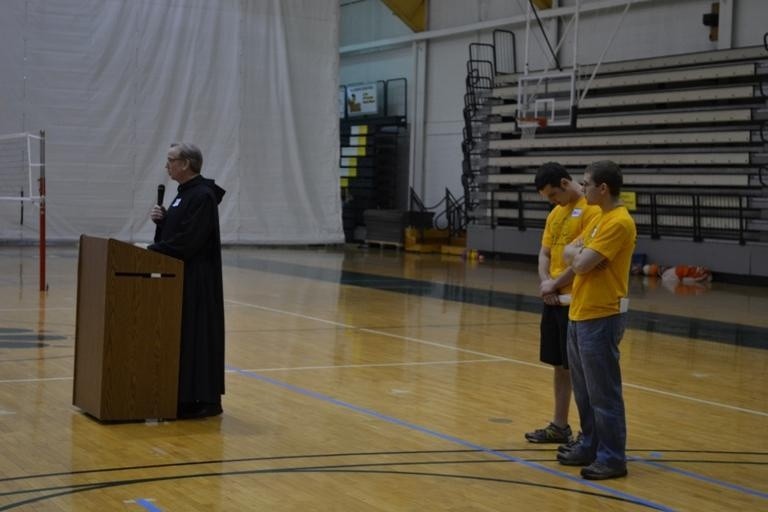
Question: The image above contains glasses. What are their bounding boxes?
[168,157,185,163]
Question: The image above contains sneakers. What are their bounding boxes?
[558,431,586,452]
[556,445,595,465]
[524,422,573,443]
[581,459,627,480]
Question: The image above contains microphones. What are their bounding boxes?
[154,184,165,224]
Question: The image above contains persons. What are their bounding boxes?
[524,161,600,453]
[134,142,227,417]
[564,159,637,478]
